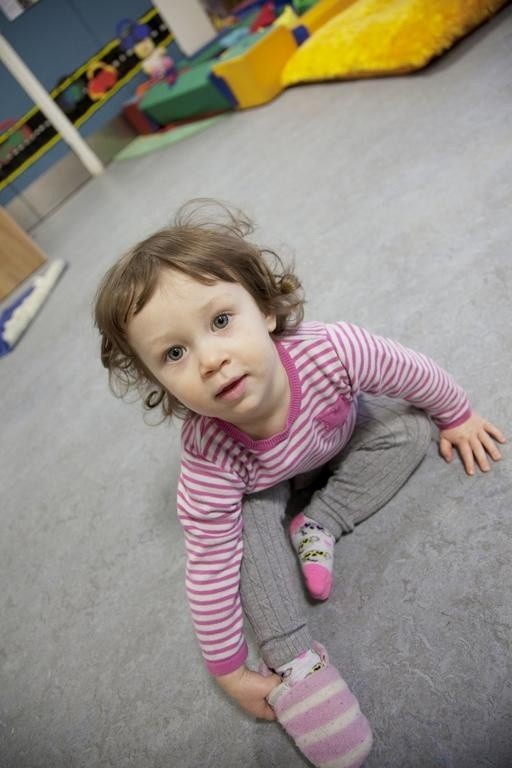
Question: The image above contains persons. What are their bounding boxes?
[83,1,358,134]
[96,196,507,768]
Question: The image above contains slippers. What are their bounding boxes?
[257,638,375,768]
